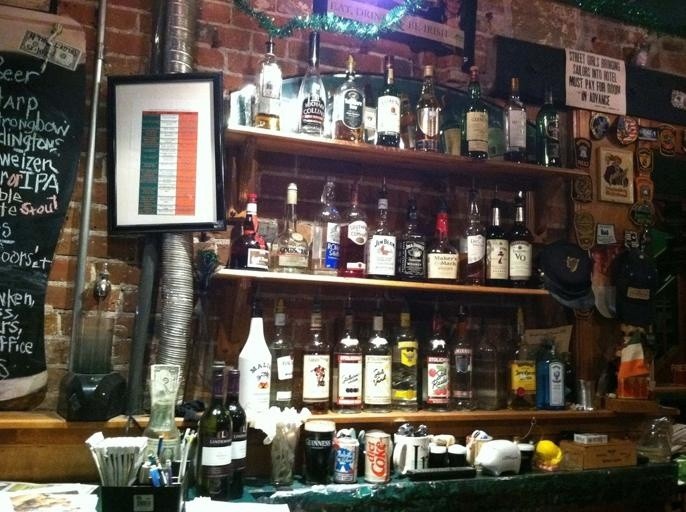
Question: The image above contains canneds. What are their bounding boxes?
[364,432,391,483]
[333,438,360,483]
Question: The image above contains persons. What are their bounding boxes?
[603,154,629,188]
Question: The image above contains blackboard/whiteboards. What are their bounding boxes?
[0,3,87,400]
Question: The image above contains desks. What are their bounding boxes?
[0,409,203,484]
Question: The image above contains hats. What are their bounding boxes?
[538,240,595,310]
[591,246,656,326]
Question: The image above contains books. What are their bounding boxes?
[84,430,150,488]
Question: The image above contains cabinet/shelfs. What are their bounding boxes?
[214,122,680,423]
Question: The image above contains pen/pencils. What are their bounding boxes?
[136,428,197,486]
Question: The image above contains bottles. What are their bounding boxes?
[426,201,460,285]
[142,382,178,461]
[224,370,248,500]
[460,66,489,159]
[439,92,462,156]
[363,298,394,414]
[269,297,295,412]
[447,445,467,468]
[397,199,426,282]
[419,299,452,412]
[199,372,232,502]
[311,175,342,276]
[450,303,474,410]
[486,198,510,288]
[458,190,487,285]
[415,64,442,153]
[534,82,562,166]
[374,53,402,147]
[392,302,419,413]
[333,296,364,415]
[508,194,532,289]
[294,31,327,136]
[474,303,579,412]
[237,304,272,417]
[231,192,269,270]
[254,38,282,130]
[362,79,377,143]
[330,51,365,143]
[398,91,417,150]
[501,75,529,161]
[366,176,398,281]
[339,175,370,278]
[293,295,333,416]
[271,183,310,274]
[517,444,535,473]
[428,446,446,469]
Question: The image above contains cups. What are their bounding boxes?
[391,433,429,477]
[302,421,335,484]
[464,436,493,465]
[267,423,299,488]
[638,417,672,463]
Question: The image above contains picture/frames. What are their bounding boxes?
[106,70,227,236]
[314,0,477,56]
